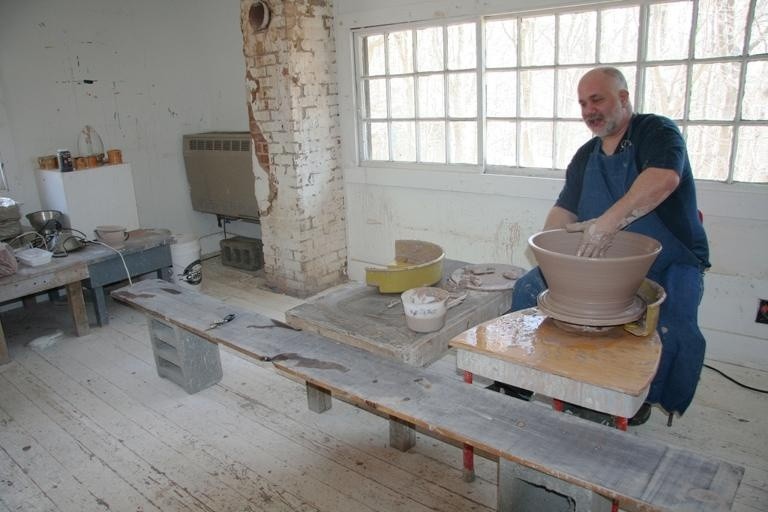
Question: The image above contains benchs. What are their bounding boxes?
[110,279,746,512]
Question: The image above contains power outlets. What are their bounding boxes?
[755,299,768,325]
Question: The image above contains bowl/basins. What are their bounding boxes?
[528,228,662,311]
[401,287,449,333]
[26,210,63,224]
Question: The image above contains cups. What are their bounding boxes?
[108,149,121,165]
[38,157,47,169]
[75,157,86,170]
[48,156,57,169]
[88,156,97,168]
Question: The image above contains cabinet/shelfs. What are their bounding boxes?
[33,165,140,245]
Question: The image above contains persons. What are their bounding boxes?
[483,66,711,428]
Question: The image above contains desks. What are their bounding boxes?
[74,229,177,328]
[285,259,513,452]
[448,306,662,512]
[0,252,91,366]
